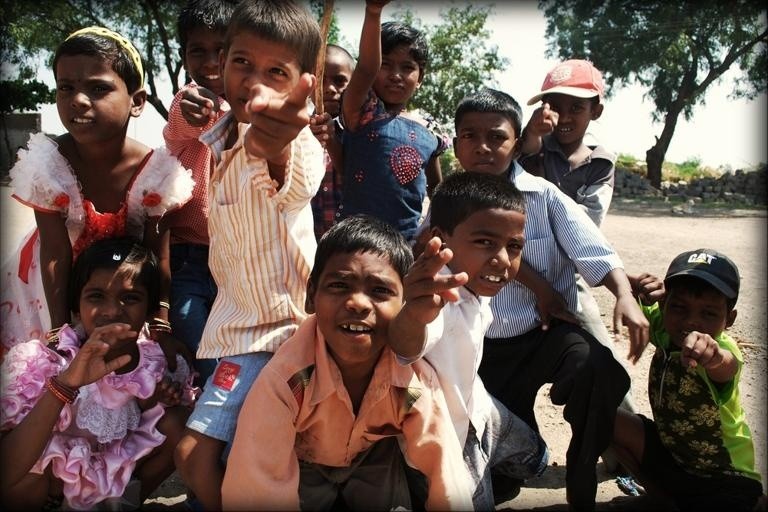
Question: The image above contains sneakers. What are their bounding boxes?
[566,452,599,506]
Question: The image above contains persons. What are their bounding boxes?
[606,249,763,511]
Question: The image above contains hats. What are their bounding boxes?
[662,246,742,307]
[525,58,604,107]
